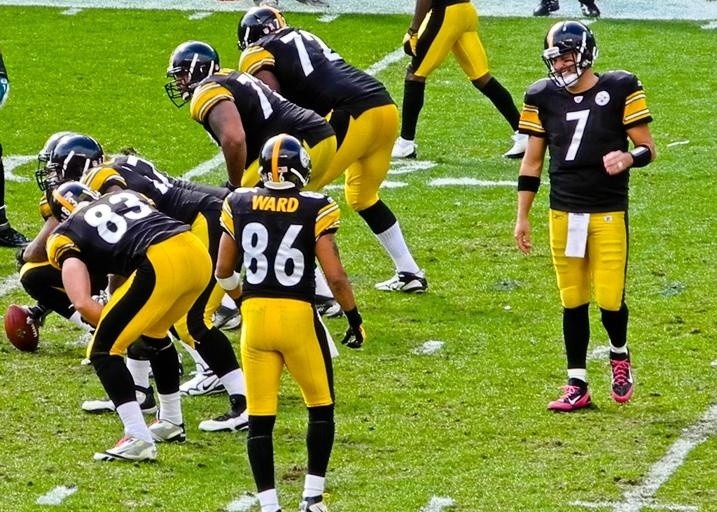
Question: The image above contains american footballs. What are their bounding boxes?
[5,305,38,351]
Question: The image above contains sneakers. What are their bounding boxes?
[178,363,227,396]
[81,385,158,415]
[391,135,418,159]
[198,394,249,432]
[375,270,428,294]
[148,410,186,444]
[298,492,330,512]
[503,130,530,159]
[0,228,30,247]
[311,294,343,319]
[533,0,600,18]
[146,354,184,378]
[547,346,634,412]
[209,304,241,330]
[93,436,157,462]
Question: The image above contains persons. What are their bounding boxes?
[37,130,233,396]
[165,41,344,321]
[515,19,655,413]
[16,136,249,434]
[1,53,28,249]
[533,0,598,17]
[238,6,428,295]
[48,169,212,460]
[214,133,366,511]
[391,1,527,158]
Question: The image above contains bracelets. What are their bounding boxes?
[518,176,540,195]
[215,271,241,292]
[629,146,652,168]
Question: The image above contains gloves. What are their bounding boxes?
[341,315,366,349]
[402,27,419,59]
[21,300,52,339]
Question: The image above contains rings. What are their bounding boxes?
[617,162,627,170]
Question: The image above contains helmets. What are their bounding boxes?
[163,5,286,108]
[541,20,600,89]
[35,131,312,225]
